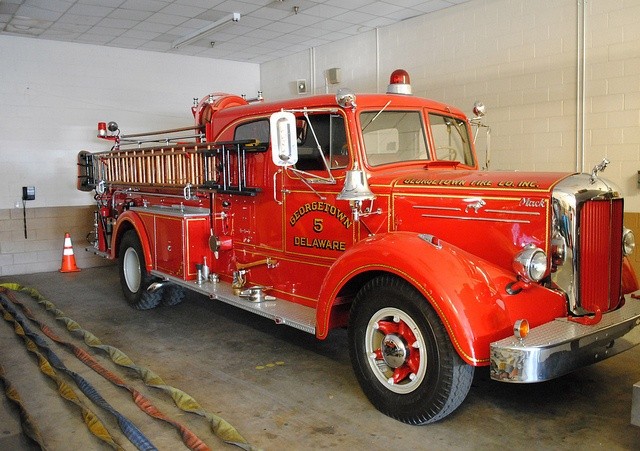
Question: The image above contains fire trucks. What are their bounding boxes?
[76,70,640,428]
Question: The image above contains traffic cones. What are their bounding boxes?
[58,233,82,274]
